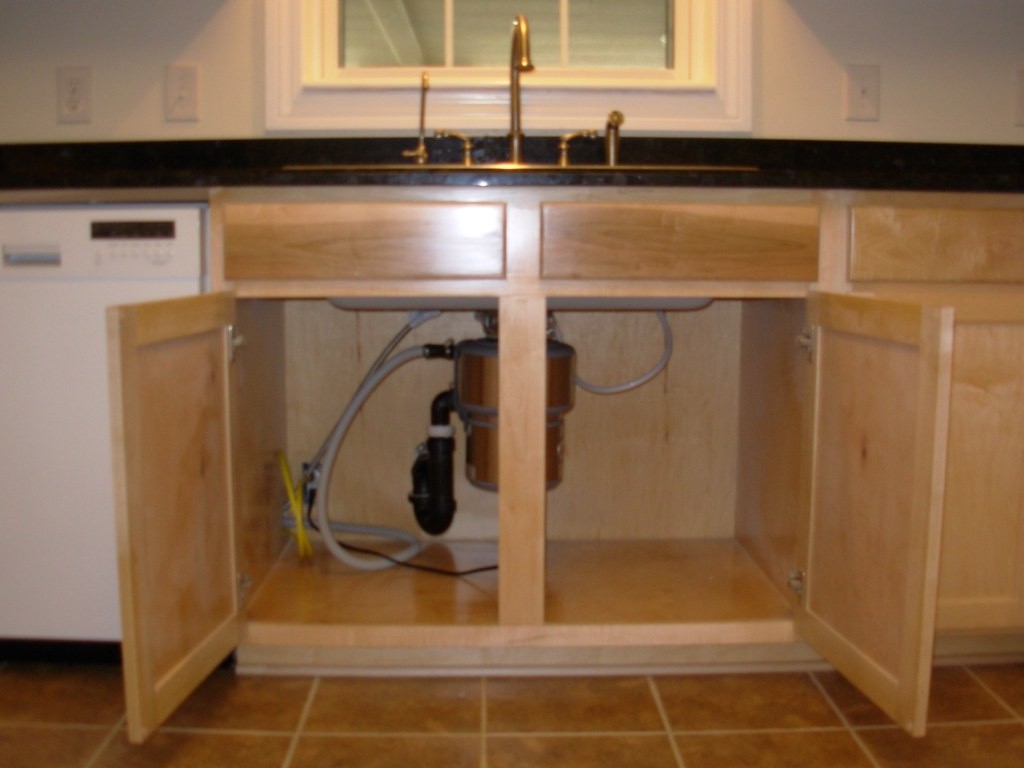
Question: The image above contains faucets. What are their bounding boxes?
[508,13,535,169]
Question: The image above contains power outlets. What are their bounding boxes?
[843,65,879,120]
[56,64,94,122]
[163,63,200,120]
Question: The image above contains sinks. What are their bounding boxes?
[285,163,761,173]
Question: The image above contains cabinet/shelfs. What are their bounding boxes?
[101,187,1024,749]
